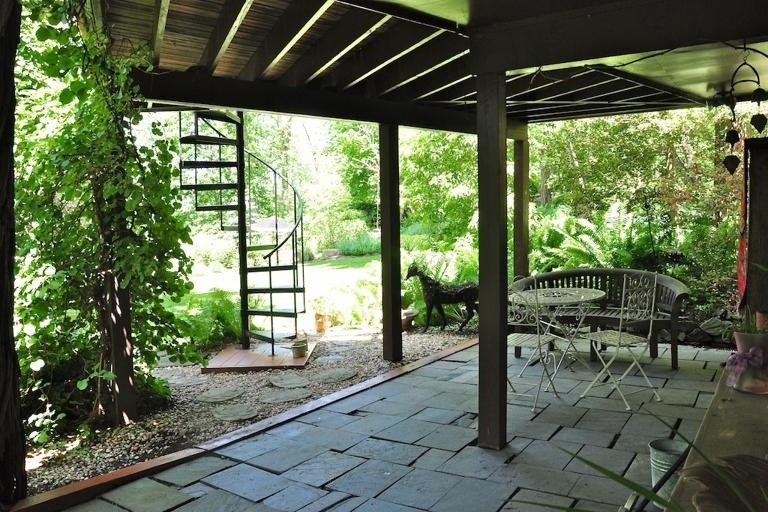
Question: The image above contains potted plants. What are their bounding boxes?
[733,304,768,365]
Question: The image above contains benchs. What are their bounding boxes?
[508,268,690,369]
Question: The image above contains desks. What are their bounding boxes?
[664,356,768,512]
[508,289,605,392]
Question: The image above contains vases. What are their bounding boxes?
[401,309,419,332]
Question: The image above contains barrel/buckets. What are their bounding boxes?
[294,338,308,351]
[647,438,690,510]
[291,342,306,358]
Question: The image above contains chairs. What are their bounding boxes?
[506,274,561,413]
[579,269,662,411]
